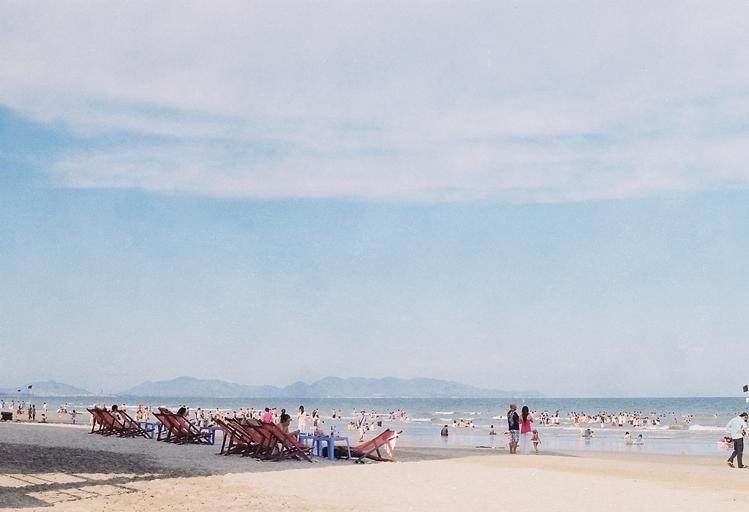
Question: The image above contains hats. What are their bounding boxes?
[739,413,748,417]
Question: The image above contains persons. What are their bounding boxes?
[489,404,560,453]
[570,410,693,443]
[721,413,749,468]
[90,403,284,439]
[440,419,473,437]
[1,401,83,424]
[278,406,407,450]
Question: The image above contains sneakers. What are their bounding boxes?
[727,461,749,469]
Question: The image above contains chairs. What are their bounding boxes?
[212,414,352,465]
[337,428,399,464]
[88,408,158,441]
[152,408,216,445]
